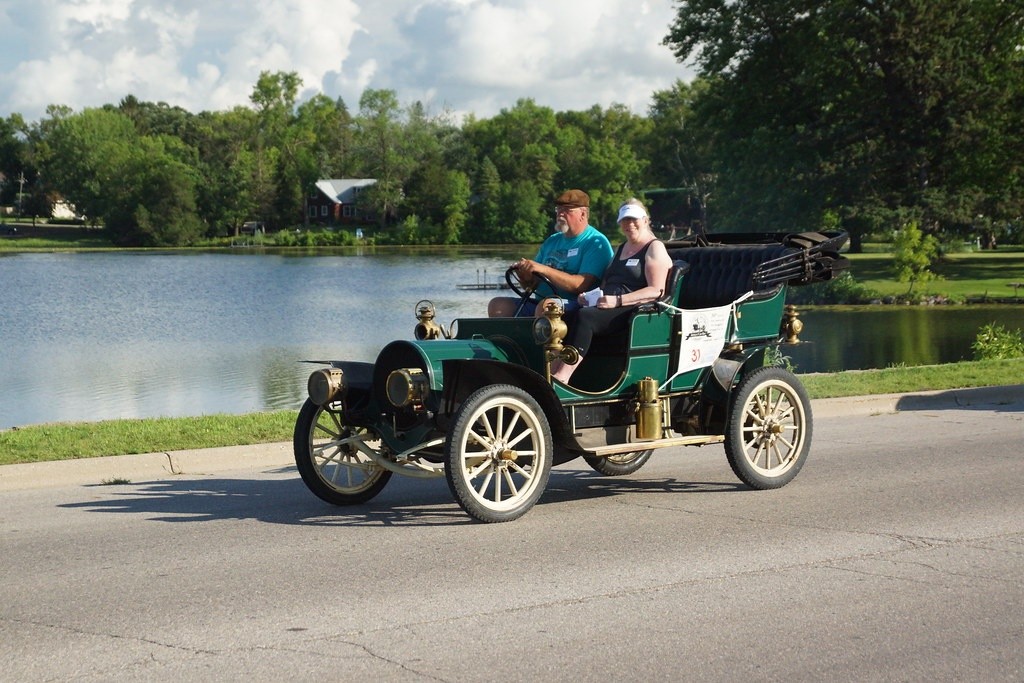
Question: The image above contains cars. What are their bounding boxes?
[289,226,851,525]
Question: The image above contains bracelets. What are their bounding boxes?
[616,295,621,307]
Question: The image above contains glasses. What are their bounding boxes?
[554,207,585,213]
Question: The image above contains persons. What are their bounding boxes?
[489,189,614,319]
[538,198,672,387]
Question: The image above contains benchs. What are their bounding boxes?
[590,259,687,389]
[666,242,784,311]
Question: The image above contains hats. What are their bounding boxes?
[553,189,590,207]
[616,205,646,223]
